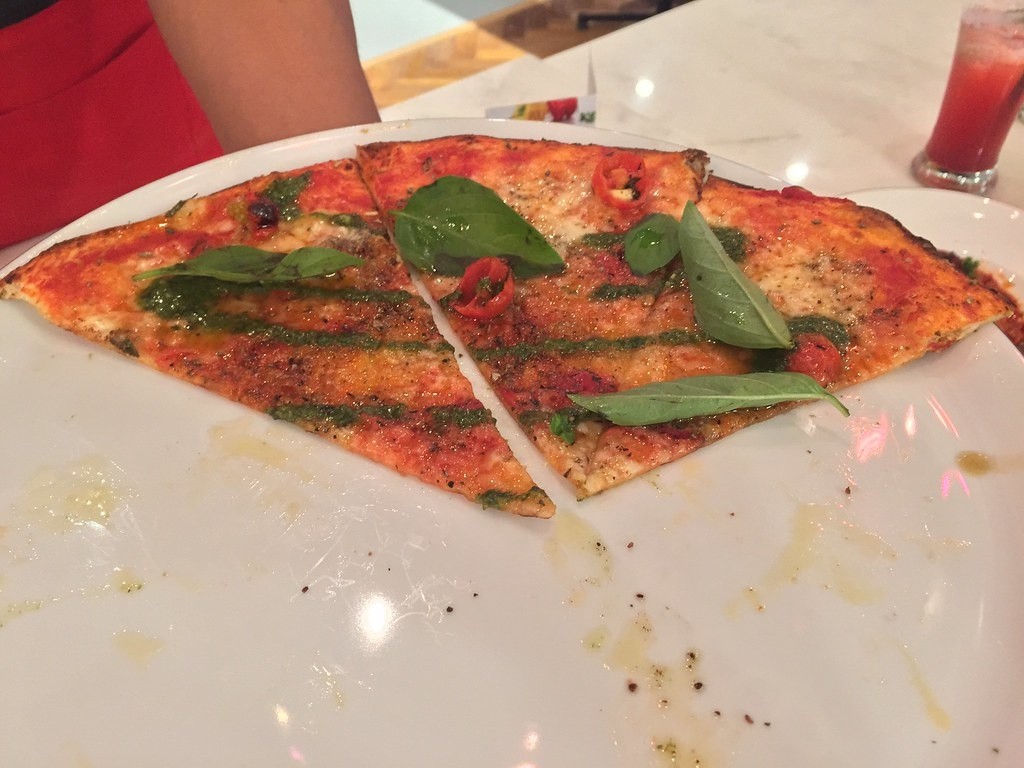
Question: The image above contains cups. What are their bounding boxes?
[912,1,1023,198]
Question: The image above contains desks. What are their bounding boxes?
[374,0,1024,354]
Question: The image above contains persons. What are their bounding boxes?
[1,1,382,248]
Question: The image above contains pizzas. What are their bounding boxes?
[0,132,1019,518]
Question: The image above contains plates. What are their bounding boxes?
[3,118,1024,768]
[827,189,1024,313]
